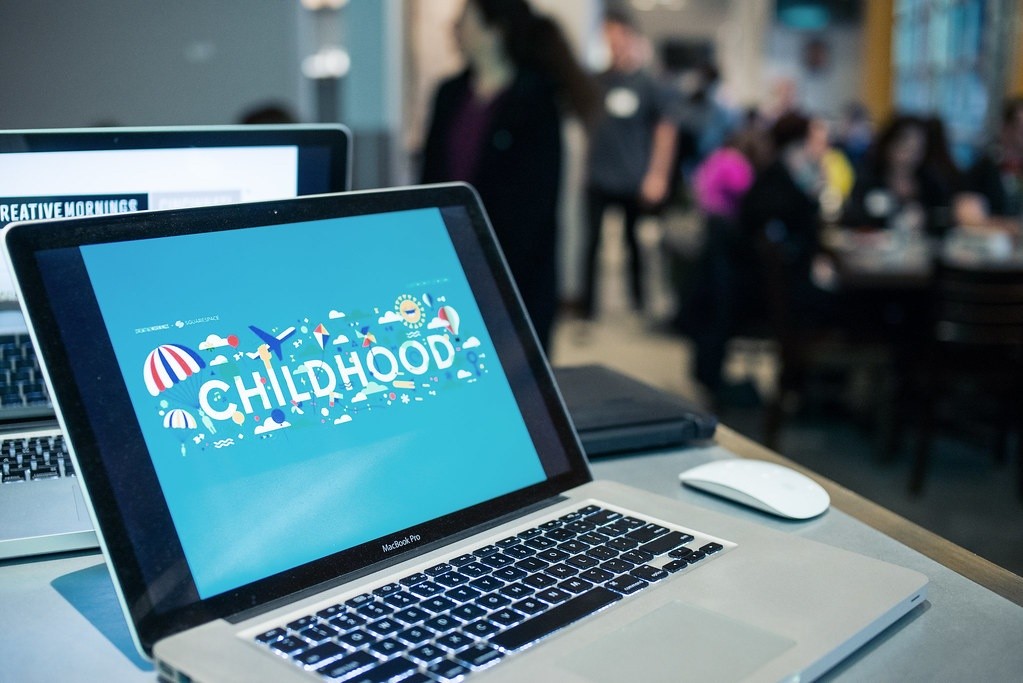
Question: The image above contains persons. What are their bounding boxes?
[411,0,567,363]
[671,65,1022,433]
[558,7,683,320]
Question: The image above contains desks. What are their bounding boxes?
[0,358,1023,683]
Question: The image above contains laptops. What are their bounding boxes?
[0,121,353,561]
[1,183,928,683]
[552,362,717,456]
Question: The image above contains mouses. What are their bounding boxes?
[678,457,831,522]
